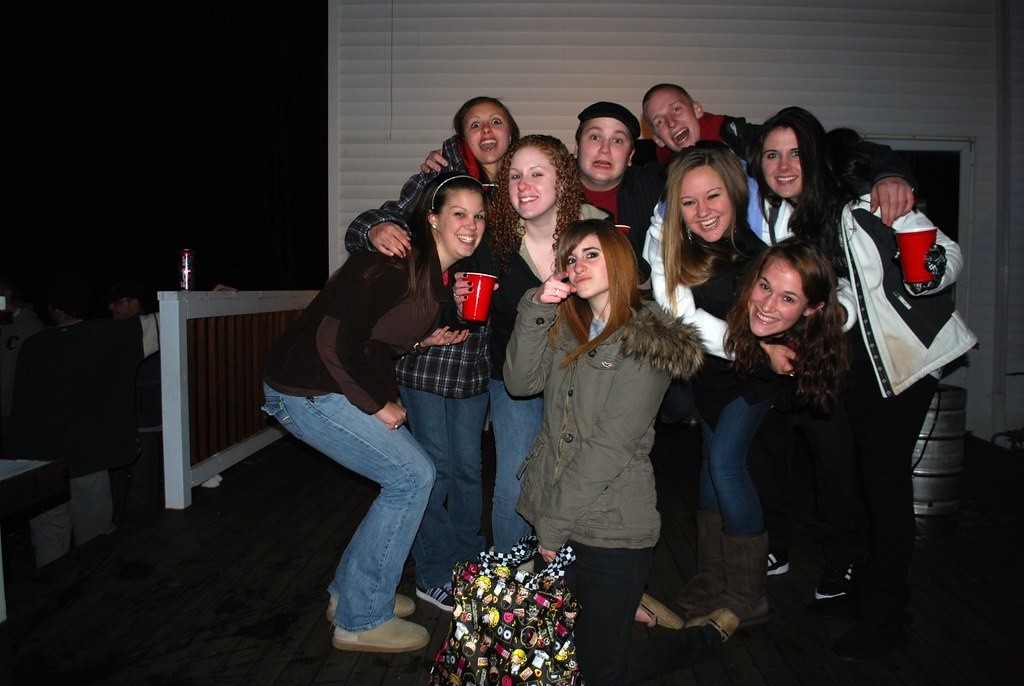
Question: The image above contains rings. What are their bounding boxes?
[790,372,794,376]
[446,343,450,345]
[395,425,399,428]
[556,289,559,296]
[454,293,457,297]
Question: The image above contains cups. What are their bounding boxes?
[461,272,498,323]
[894,227,938,282]
[615,225,631,235]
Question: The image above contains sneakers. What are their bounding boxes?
[767,553,790,574]
[416,580,453,611]
[815,562,856,600]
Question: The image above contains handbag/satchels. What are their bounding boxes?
[427,535,585,686]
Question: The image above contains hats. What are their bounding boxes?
[578,101,641,141]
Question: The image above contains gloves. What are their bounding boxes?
[895,243,947,293]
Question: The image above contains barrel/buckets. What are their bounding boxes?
[910,384,968,517]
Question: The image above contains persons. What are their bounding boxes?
[0,279,239,569]
[262,172,486,653]
[422,100,672,301]
[346,98,523,613]
[643,83,915,227]
[504,219,740,686]
[750,107,978,660]
[641,149,860,627]
[486,133,613,552]
[722,237,861,602]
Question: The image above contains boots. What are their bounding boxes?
[684,528,773,624]
[675,510,728,608]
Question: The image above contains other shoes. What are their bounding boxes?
[327,591,416,622]
[333,615,429,654]
[831,623,907,659]
[806,593,866,618]
[639,593,683,630]
[687,607,740,641]
